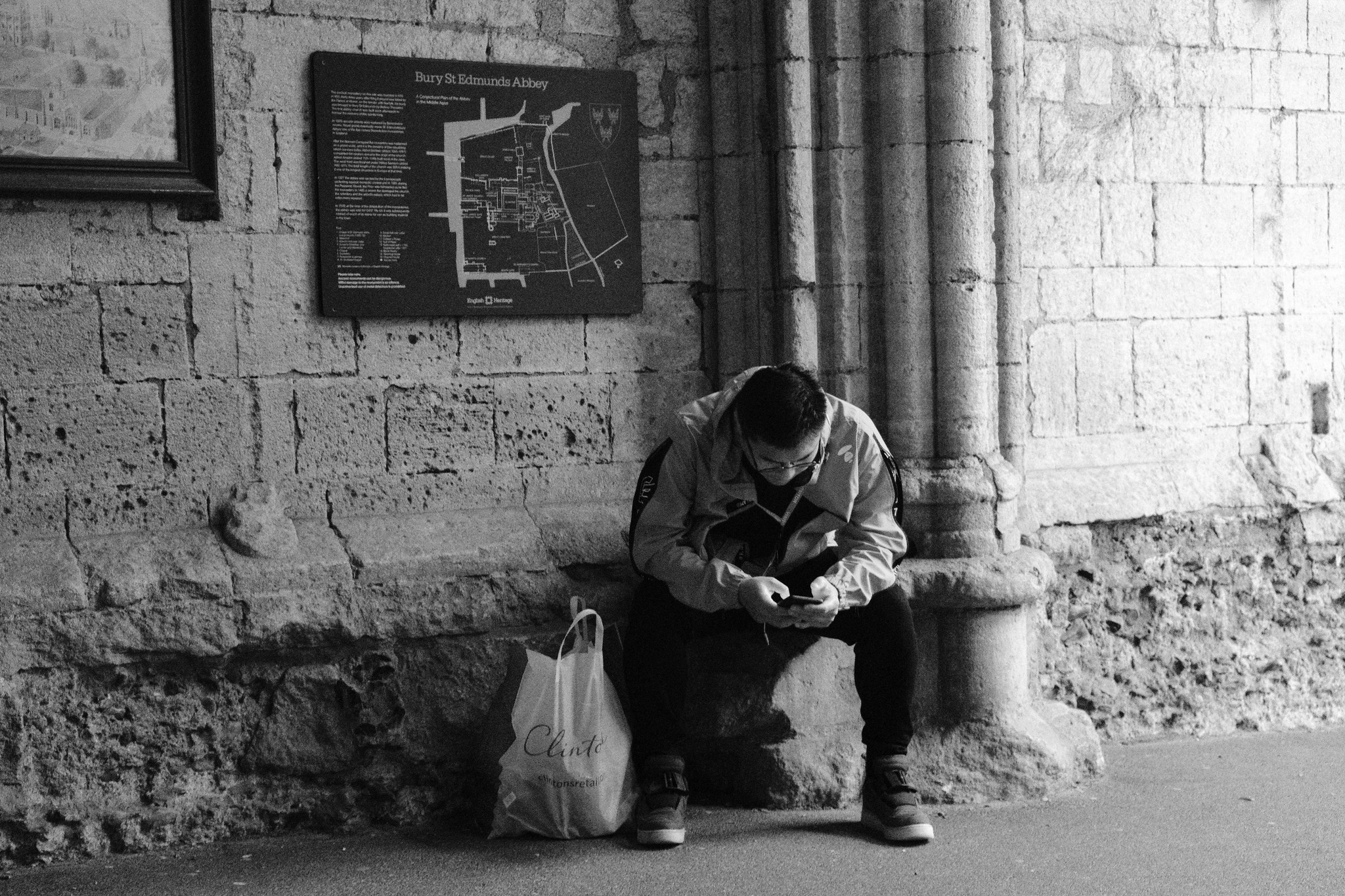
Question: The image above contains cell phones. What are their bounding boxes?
[777,596,823,608]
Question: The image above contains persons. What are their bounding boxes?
[619,362,935,844]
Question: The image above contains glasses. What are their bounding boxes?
[742,437,822,475]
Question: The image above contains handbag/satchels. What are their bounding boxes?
[485,597,642,841]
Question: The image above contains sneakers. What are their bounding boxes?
[859,766,935,842]
[633,754,690,847]
